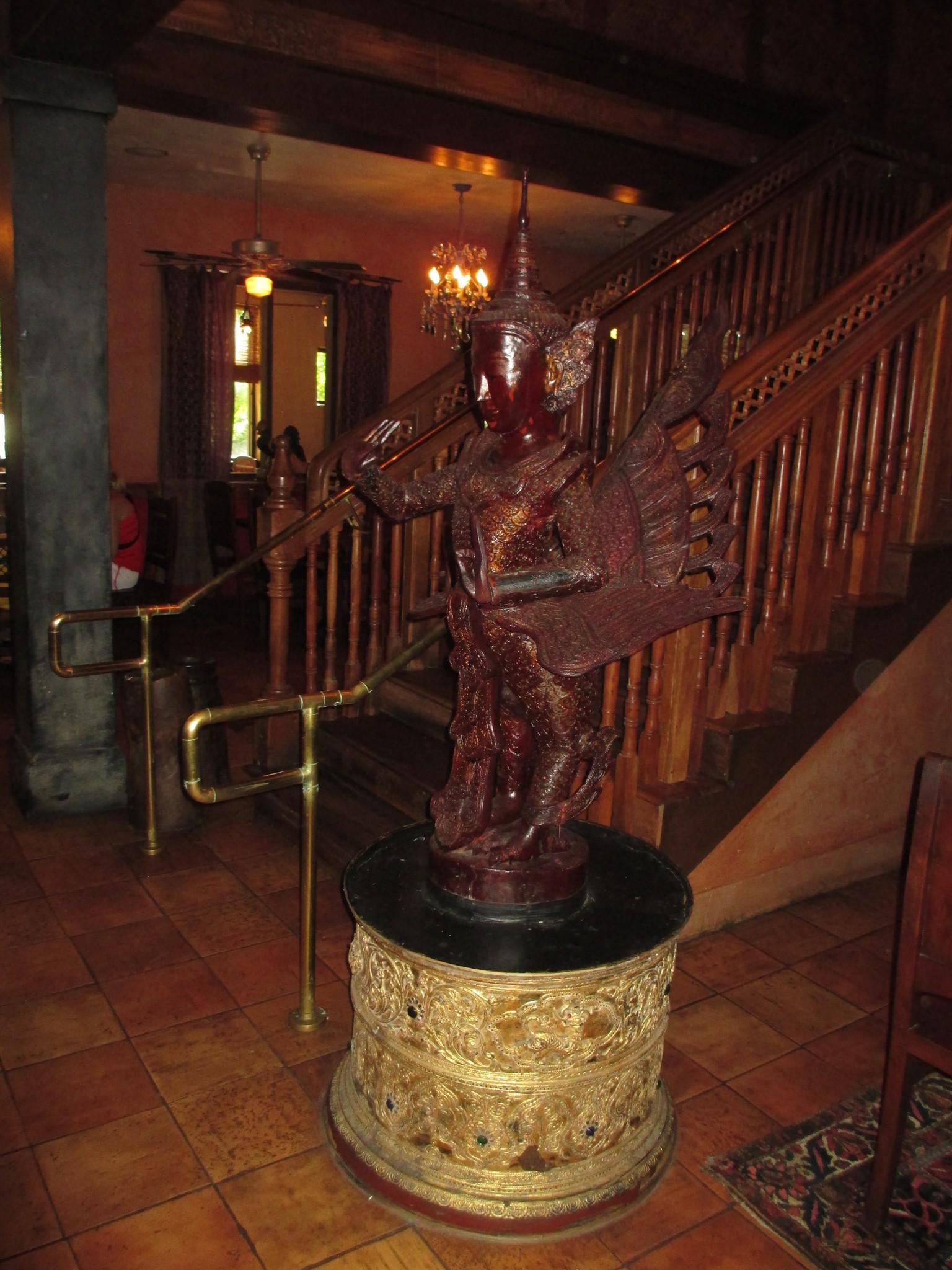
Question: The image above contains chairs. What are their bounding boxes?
[203,478,269,641]
[860,750,952,1240]
[111,482,179,666]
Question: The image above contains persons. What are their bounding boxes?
[110,487,141,589]
[340,288,607,859]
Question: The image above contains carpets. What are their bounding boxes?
[701,1060,952,1270]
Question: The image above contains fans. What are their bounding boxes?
[139,145,403,295]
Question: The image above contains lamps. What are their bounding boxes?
[243,268,274,299]
[419,183,490,353]
